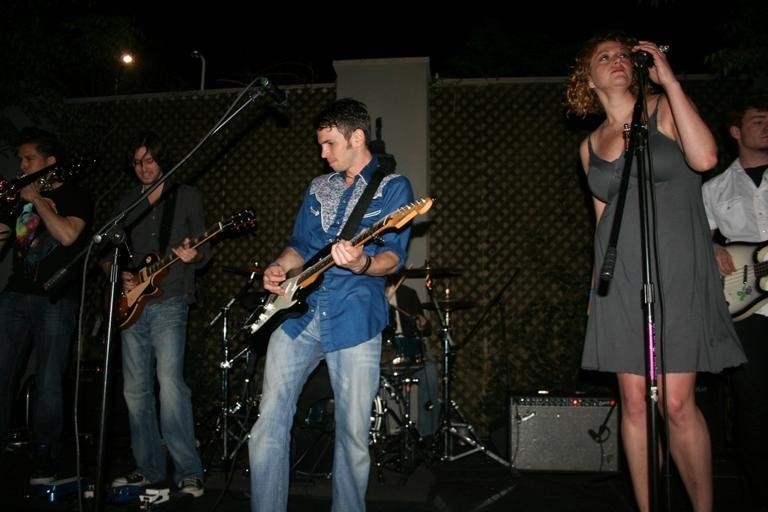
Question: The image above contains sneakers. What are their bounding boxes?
[29,472,81,486]
[112,471,151,487]
[177,478,204,497]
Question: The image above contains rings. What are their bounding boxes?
[656,43,671,56]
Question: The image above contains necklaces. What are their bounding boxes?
[603,109,634,135]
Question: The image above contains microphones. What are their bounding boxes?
[261,78,291,108]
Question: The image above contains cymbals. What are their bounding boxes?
[422,300,477,310]
[402,268,461,279]
[223,266,265,275]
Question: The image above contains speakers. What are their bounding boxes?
[507,393,621,476]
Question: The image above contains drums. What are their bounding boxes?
[379,379,419,434]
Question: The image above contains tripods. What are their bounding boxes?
[194,308,252,474]
[421,308,512,475]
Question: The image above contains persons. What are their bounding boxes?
[97,133,216,497]
[563,28,750,511]
[383,275,432,360]
[1,126,87,488]
[702,95,768,512]
[244,95,413,510]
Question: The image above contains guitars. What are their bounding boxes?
[104,211,257,331]
[719,241,768,322]
[248,197,433,334]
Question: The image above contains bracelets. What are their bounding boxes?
[353,253,372,276]
